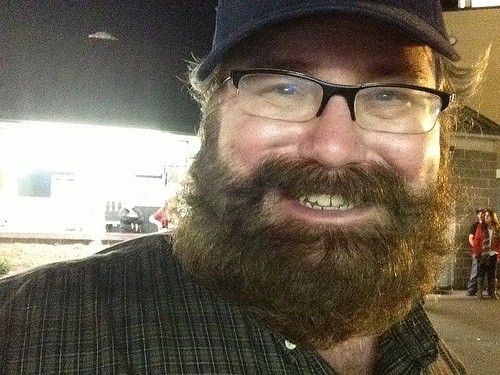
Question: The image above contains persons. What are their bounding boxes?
[468,208,499,300]
[0,0,469,375]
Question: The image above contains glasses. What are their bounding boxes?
[220,68,457,134]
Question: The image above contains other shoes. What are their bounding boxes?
[477,294,483,299]
[488,293,495,298]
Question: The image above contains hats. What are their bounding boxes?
[476,207,484,212]
[196,0,462,83]
